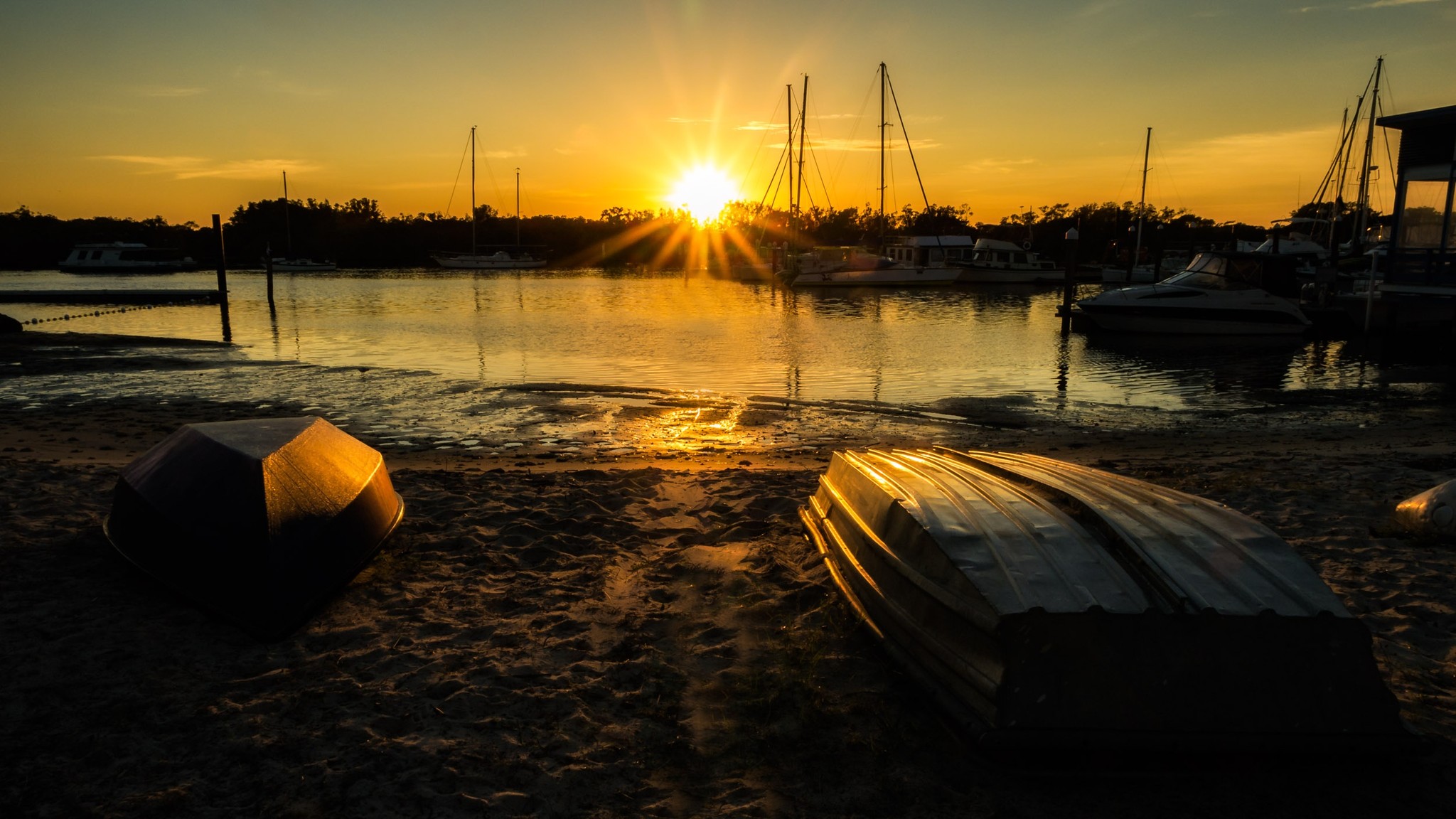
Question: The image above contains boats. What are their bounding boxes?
[1066,250,1315,341]
[57,242,198,275]
[798,443,1403,765]
[102,417,407,609]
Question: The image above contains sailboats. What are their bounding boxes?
[712,61,1070,289]
[260,170,336,271]
[1078,56,1439,300]
[429,127,547,268]
